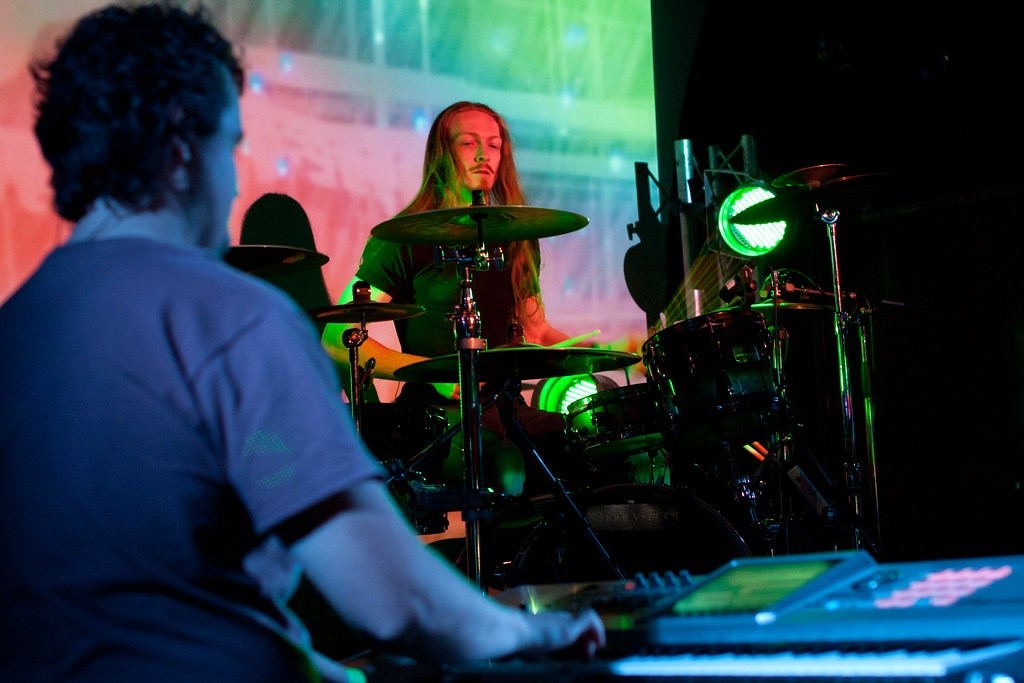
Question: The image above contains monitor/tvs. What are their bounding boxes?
[671,559,840,613]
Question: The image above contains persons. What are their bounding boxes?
[321,101,599,513]
[0,1,606,683]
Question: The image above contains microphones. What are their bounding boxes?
[759,269,787,298]
[719,261,756,303]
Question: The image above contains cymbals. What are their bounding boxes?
[370,203,589,244]
[391,342,643,390]
[306,300,425,324]
[729,162,888,226]
[222,244,330,270]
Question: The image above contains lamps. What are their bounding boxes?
[539,375,621,436]
[718,179,787,258]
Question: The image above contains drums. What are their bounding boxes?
[567,382,662,456]
[549,430,672,486]
[497,479,751,585]
[642,308,784,448]
